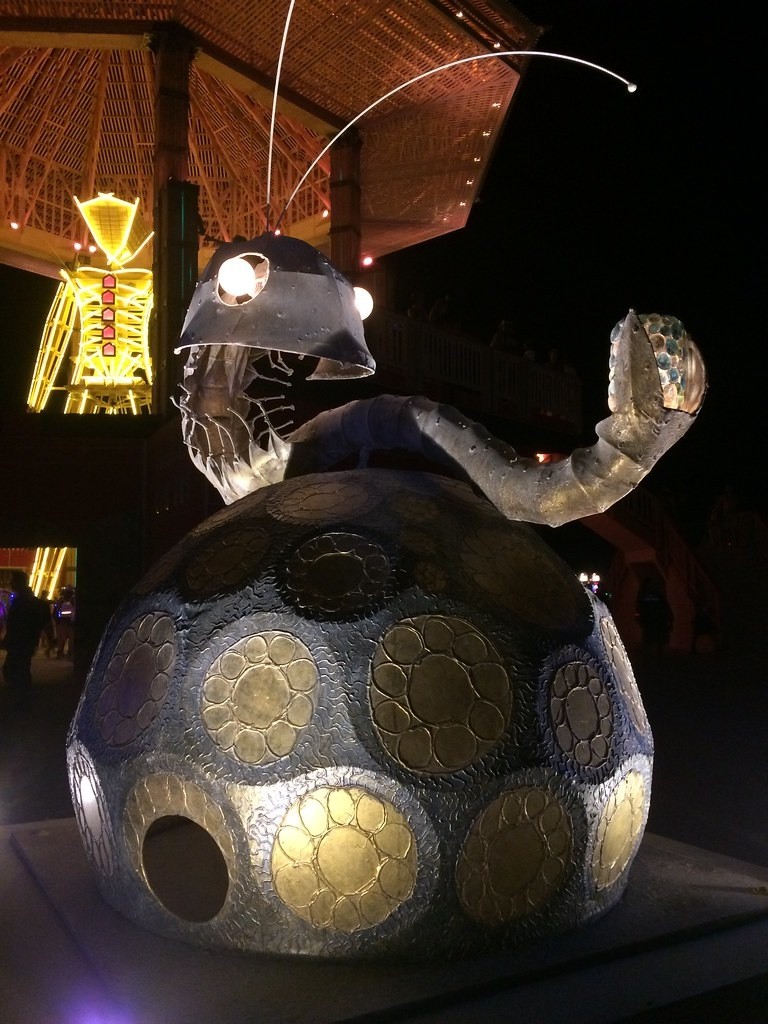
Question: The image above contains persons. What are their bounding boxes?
[0,570,75,691]
